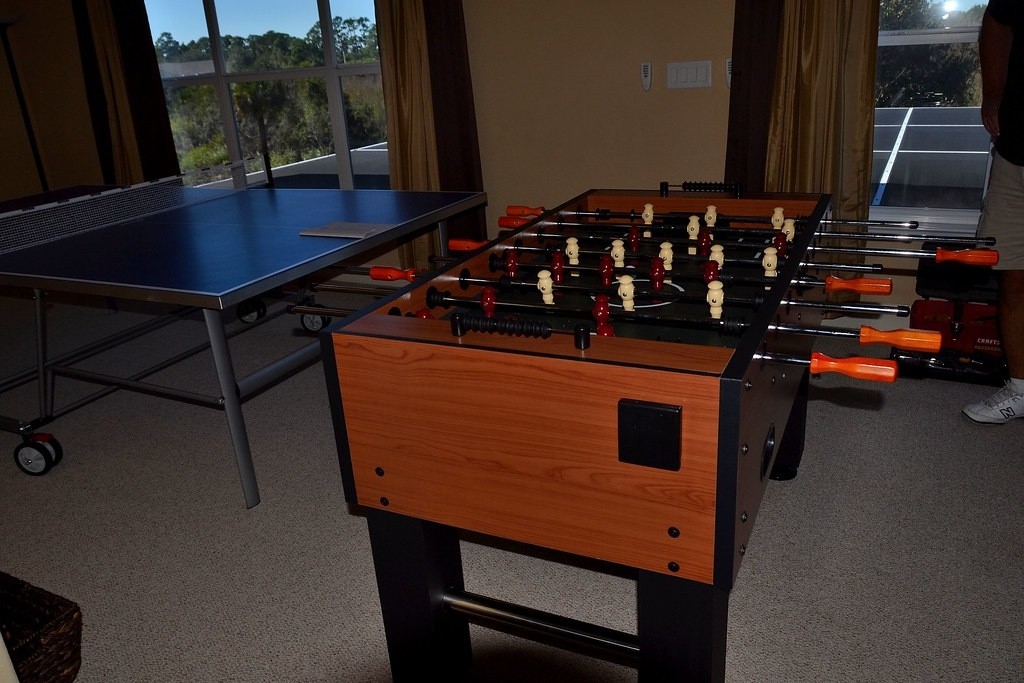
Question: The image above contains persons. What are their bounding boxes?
[962,0,1024,423]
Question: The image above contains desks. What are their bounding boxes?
[0,183,489,510]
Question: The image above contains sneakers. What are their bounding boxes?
[961,380,1024,424]
